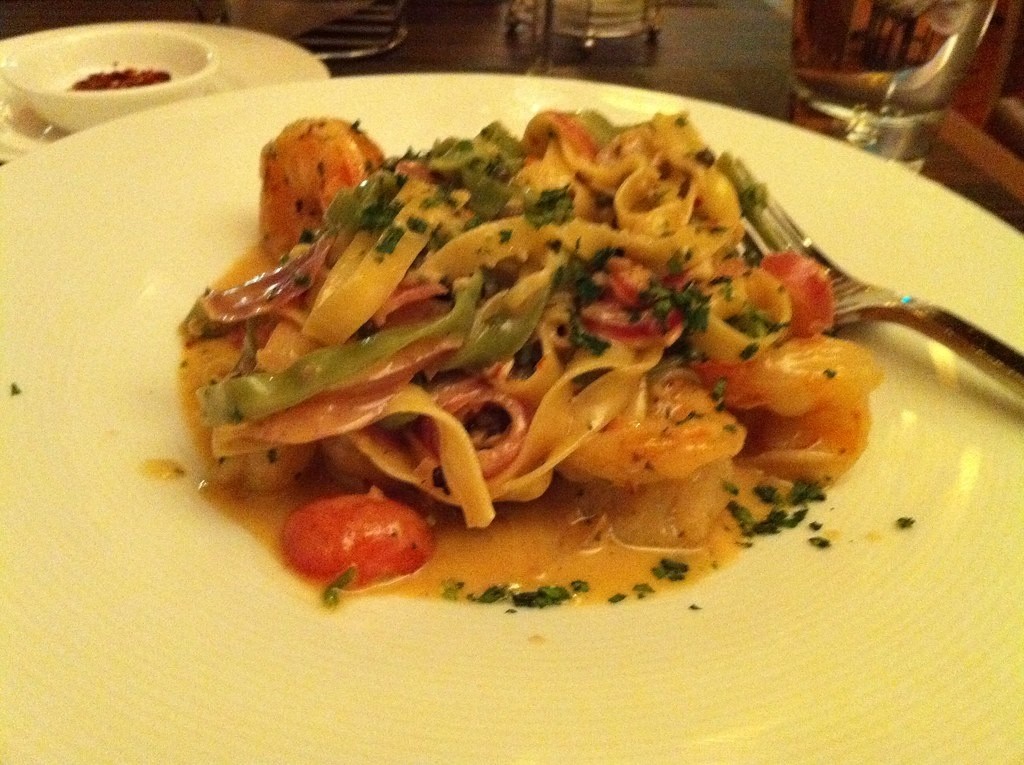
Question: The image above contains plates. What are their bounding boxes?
[0,20,332,164]
[0,73,1024,765]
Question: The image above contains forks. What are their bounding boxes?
[741,197,1024,396]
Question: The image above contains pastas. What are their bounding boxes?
[179,106,883,608]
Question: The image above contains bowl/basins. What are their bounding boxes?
[5,29,221,133]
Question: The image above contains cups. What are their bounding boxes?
[789,0,997,125]
